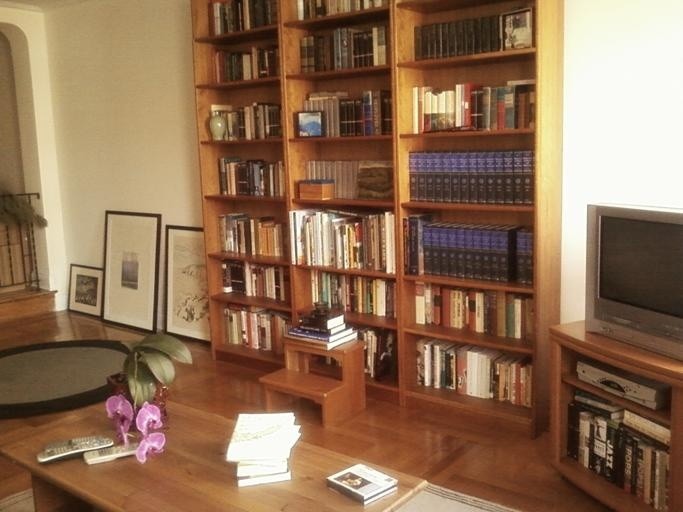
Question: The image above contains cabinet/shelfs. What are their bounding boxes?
[253,334,367,429]
[390,1,565,436]
[279,0,398,409]
[543,321,683,511]
[189,1,289,365]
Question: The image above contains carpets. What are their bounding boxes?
[0,339,132,420]
[0,393,466,512]
[0,483,526,512]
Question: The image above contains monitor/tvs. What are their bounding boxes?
[586,204,683,361]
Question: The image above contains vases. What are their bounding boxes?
[106,372,168,425]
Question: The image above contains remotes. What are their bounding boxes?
[84,443,137,464]
[37,437,114,462]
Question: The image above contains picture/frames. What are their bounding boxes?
[67,263,104,318]
[164,224,211,345]
[100,211,162,336]
[499,7,535,51]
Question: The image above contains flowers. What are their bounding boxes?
[105,335,192,464]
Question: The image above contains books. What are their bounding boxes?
[413,79,536,134]
[326,463,398,506]
[414,14,502,61]
[288,208,396,275]
[408,150,534,205]
[224,303,292,355]
[208,0,276,35]
[309,269,397,320]
[219,157,285,196]
[210,47,279,84]
[281,310,360,351]
[210,103,281,140]
[414,282,534,345]
[226,411,302,488]
[415,338,532,408]
[217,213,286,258]
[300,28,388,74]
[566,389,671,510]
[303,90,391,137]
[352,326,396,381]
[222,259,290,305]
[306,159,392,199]
[296,0,389,20]
[402,214,534,285]
